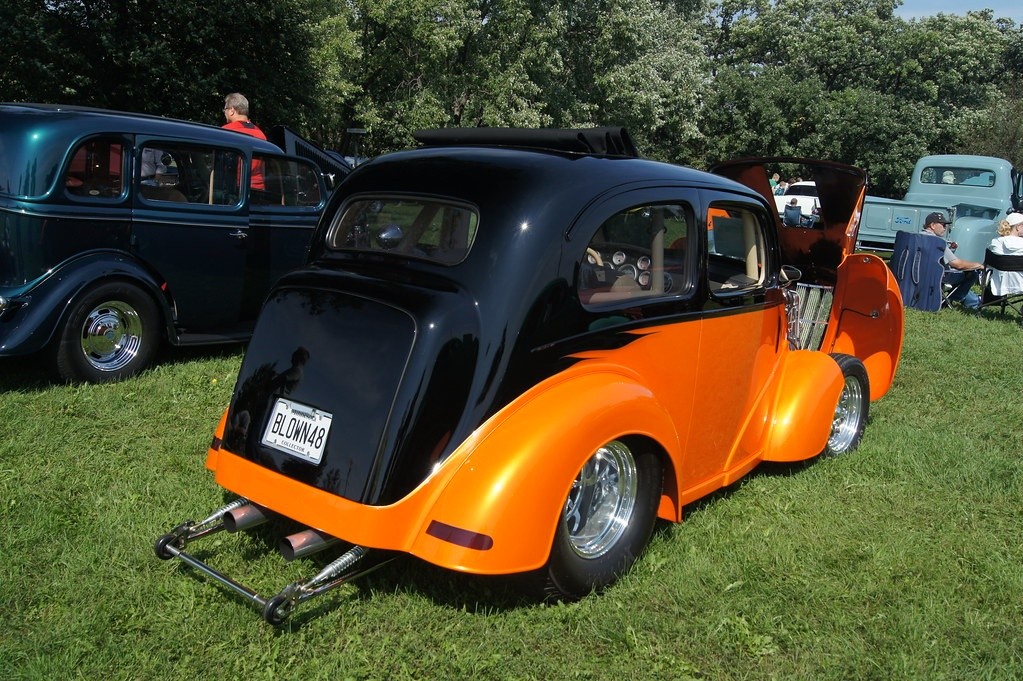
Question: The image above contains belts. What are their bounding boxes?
[140,175,155,181]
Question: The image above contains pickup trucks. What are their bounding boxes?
[855,154,1015,274]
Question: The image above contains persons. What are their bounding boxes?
[220,93,268,190]
[918,211,984,309]
[982,212,1023,315]
[131,143,169,188]
[783,198,825,228]
[66,174,113,198]
[769,173,803,196]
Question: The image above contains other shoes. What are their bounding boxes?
[1018,306,1023,316]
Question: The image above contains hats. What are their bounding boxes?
[925,212,954,225]
[1005,212,1023,226]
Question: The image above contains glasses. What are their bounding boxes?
[222,107,233,113]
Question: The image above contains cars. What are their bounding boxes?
[774,179,824,219]
[0,103,337,388]
[214,141,907,590]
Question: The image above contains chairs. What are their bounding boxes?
[975,247,1023,319]
[888,230,983,313]
